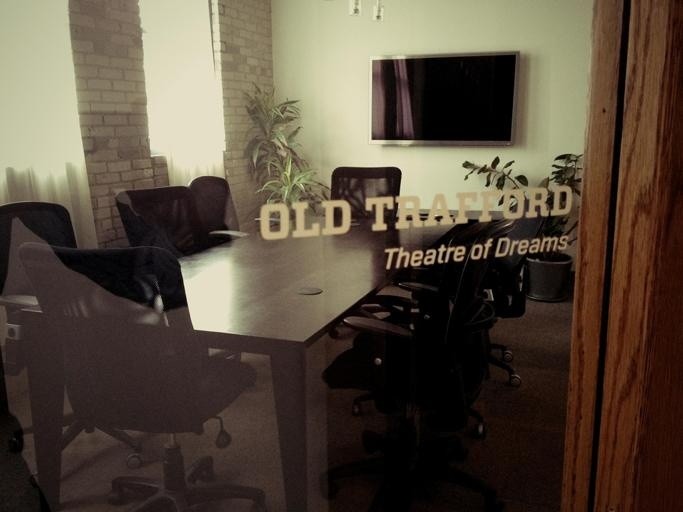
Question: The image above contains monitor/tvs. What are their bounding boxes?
[368,50,520,146]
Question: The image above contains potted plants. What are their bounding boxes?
[461,153,584,303]
